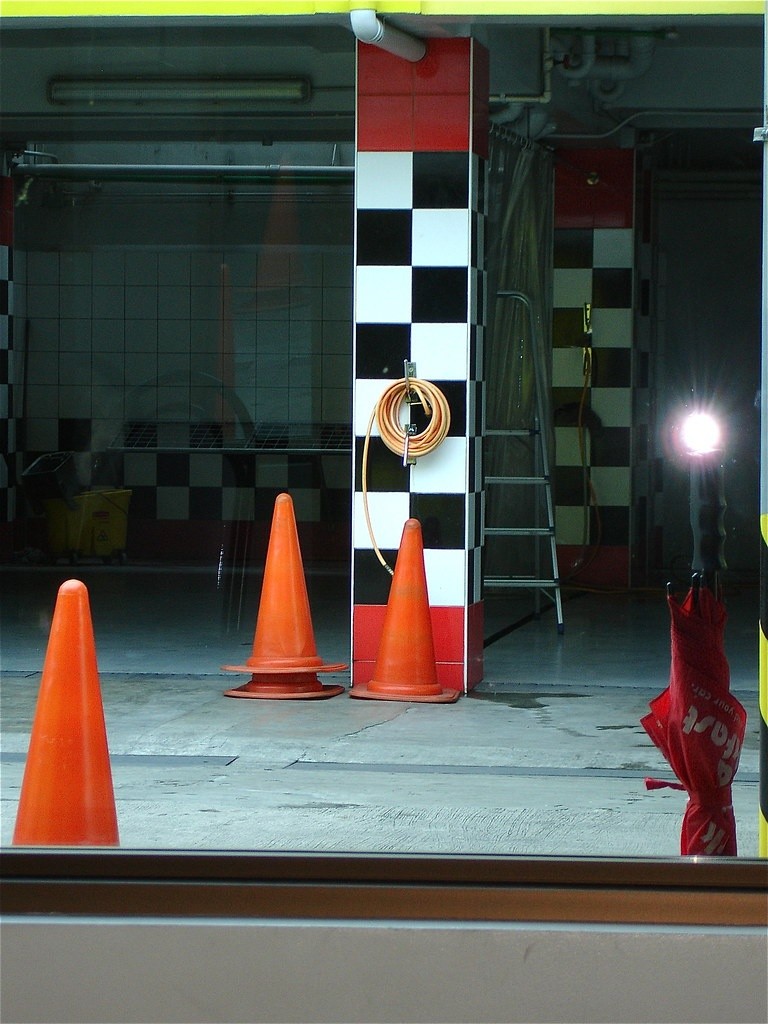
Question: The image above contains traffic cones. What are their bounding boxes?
[8,578,120,849]
[349,516,464,706]
[219,493,348,701]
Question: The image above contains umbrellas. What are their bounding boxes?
[644,433,747,857]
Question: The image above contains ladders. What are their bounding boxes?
[484,291,564,635]
